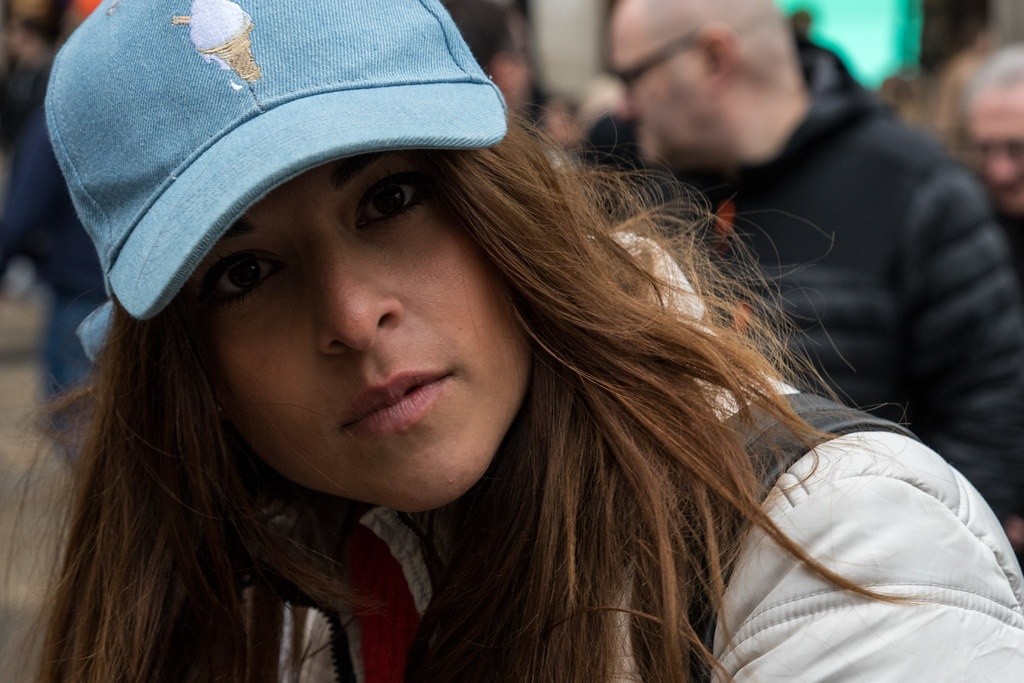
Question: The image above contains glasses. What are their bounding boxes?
[608,39,697,102]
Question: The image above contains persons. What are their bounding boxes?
[0,0,1024,683]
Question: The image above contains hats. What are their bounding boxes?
[46,0,507,322]
